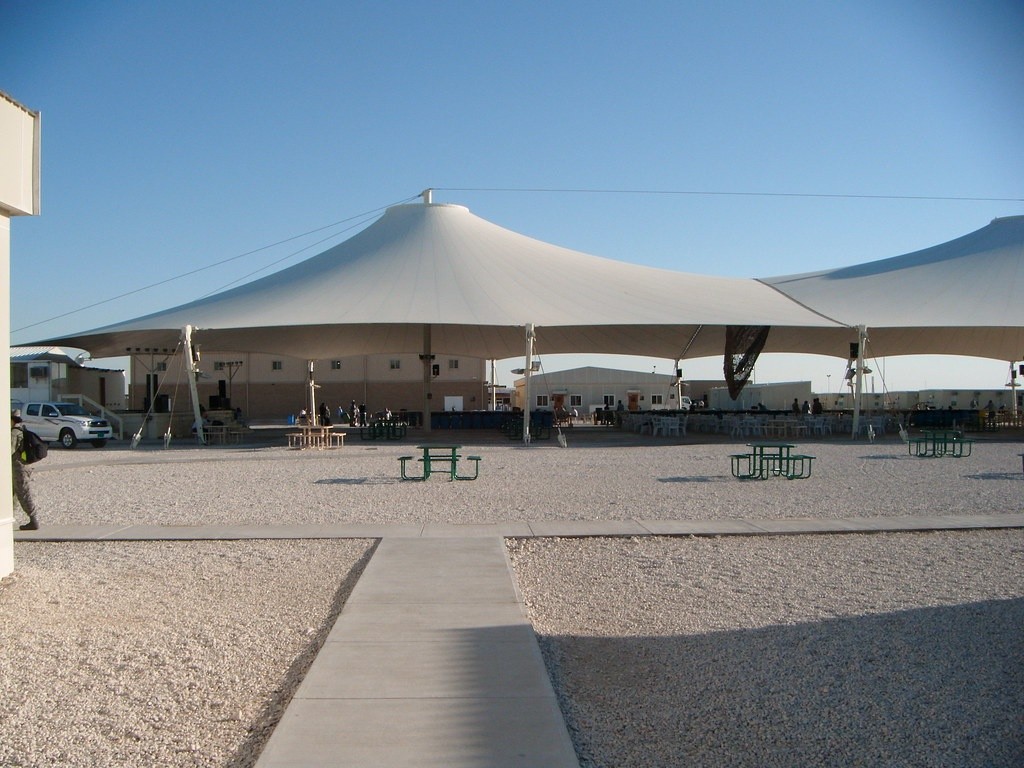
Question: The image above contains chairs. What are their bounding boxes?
[593,408,1024,438]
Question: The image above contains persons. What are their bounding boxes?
[384,408,392,420]
[757,403,766,410]
[700,400,704,408]
[349,399,366,427]
[299,406,307,418]
[916,404,920,410]
[984,399,996,427]
[191,410,216,446]
[604,403,609,410]
[617,400,624,411]
[802,401,810,413]
[926,404,930,410]
[319,403,331,426]
[812,398,822,414]
[571,407,578,415]
[11,408,39,530]
[998,404,1006,414]
[792,398,800,411]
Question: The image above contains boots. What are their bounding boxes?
[19,517,40,530]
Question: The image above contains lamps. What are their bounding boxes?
[845,369,856,379]
[432,364,440,379]
[511,369,525,375]
[1012,364,1024,379]
[863,366,873,374]
[669,382,679,387]
[681,383,689,387]
[530,361,541,372]
[418,354,436,363]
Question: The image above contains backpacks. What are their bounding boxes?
[11,425,47,465]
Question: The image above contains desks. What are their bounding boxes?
[298,425,334,451]
[919,429,961,458]
[369,419,398,441]
[768,419,801,439]
[747,442,796,480]
[417,442,461,483]
[203,425,242,445]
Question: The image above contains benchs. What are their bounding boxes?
[397,456,414,481]
[907,438,974,459]
[466,456,483,481]
[357,425,408,442]
[286,433,347,450]
[417,454,461,481]
[193,432,253,445]
[727,454,750,479]
[791,453,817,480]
[746,453,783,480]
[759,456,803,480]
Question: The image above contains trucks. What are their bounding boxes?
[681,396,692,412]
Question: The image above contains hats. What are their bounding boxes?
[11,408,22,417]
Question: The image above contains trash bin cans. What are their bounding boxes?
[341,412,351,423]
[287,413,295,425]
[160,395,169,413]
[209,395,220,410]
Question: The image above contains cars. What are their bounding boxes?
[17,402,114,447]
